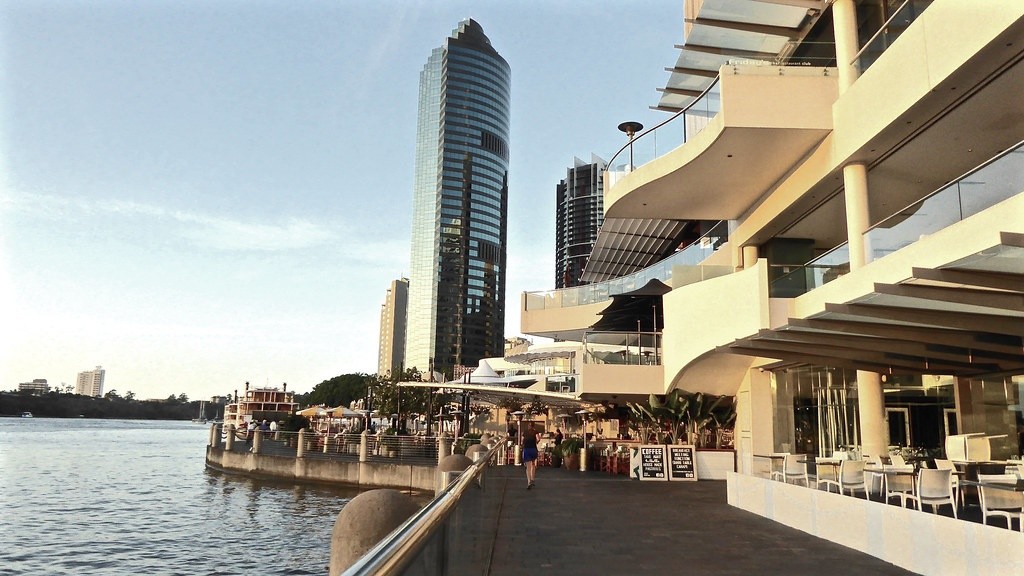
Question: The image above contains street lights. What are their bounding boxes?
[575,409,593,450]
[448,410,462,440]
[510,410,528,445]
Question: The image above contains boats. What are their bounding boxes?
[21,411,34,418]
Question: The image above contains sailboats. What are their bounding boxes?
[191,400,224,425]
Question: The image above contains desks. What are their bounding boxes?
[754,454,821,486]
[863,467,965,511]
[796,459,875,494]
[952,460,1023,518]
[641,351,654,365]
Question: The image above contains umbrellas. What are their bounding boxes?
[325,405,363,424]
[294,405,329,425]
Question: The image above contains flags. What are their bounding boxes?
[565,265,570,288]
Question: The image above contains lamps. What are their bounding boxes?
[881,368,896,384]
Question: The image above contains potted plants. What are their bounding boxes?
[552,433,593,470]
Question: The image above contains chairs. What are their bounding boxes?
[507,445,1024,536]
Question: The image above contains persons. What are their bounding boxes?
[352,424,358,432]
[506,424,518,437]
[416,432,427,436]
[549,432,556,439]
[372,421,376,431]
[309,423,322,451]
[269,420,277,439]
[521,421,540,490]
[555,429,563,446]
[248,419,268,441]
[334,423,351,452]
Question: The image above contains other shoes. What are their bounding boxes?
[530,480,536,486]
[527,483,533,490]
